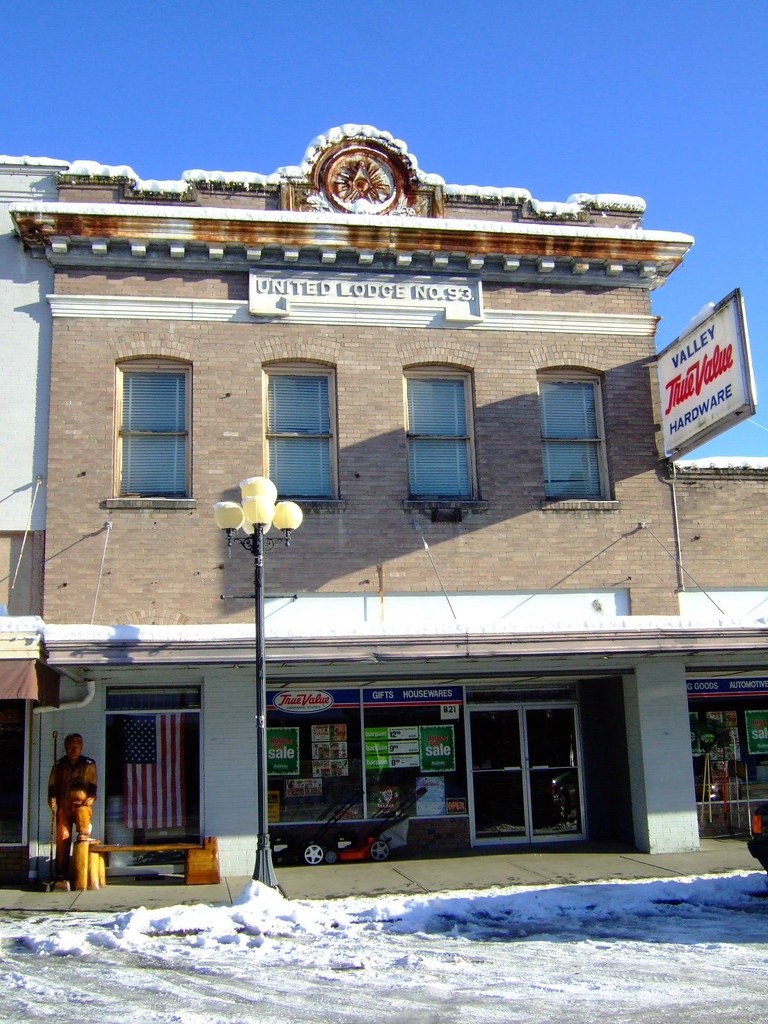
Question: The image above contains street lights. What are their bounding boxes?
[212,475,305,905]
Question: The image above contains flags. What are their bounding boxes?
[124,713,187,830]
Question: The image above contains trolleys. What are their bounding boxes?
[271,786,428,867]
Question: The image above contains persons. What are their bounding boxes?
[48,733,98,889]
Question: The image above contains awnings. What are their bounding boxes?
[0,659,62,708]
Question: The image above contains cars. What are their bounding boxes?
[747,802,768,874]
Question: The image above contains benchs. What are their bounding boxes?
[75,837,220,892]
